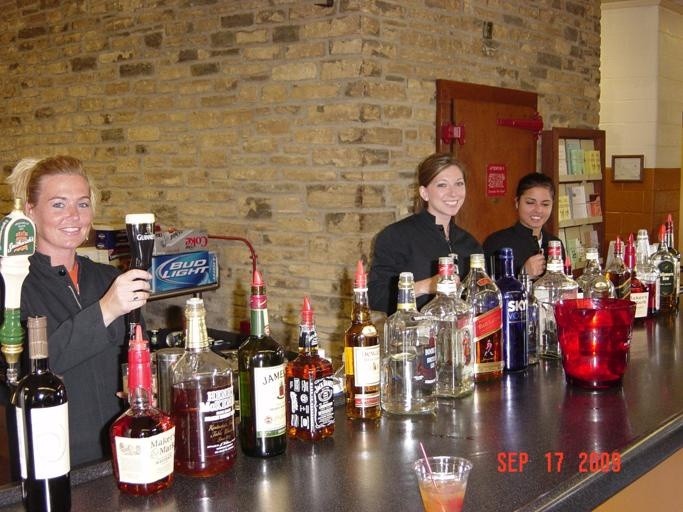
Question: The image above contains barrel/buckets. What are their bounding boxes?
[557,387,639,470]
[541,295,637,390]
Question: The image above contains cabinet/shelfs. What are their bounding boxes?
[542,128,605,271]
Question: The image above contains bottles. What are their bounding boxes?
[381,215,682,414]
[287,297,334,443]
[237,271,286,458]
[9,314,71,512]
[169,297,237,475]
[106,325,176,495]
[119,323,186,413]
[343,261,381,420]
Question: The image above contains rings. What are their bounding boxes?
[132,292,138,301]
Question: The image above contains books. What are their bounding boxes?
[558,138,602,270]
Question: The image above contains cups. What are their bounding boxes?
[125,213,154,268]
[413,456,473,512]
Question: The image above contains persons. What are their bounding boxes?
[482,172,566,281]
[366,154,485,318]
[0,154,153,470]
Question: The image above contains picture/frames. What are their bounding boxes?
[610,154,643,183]
